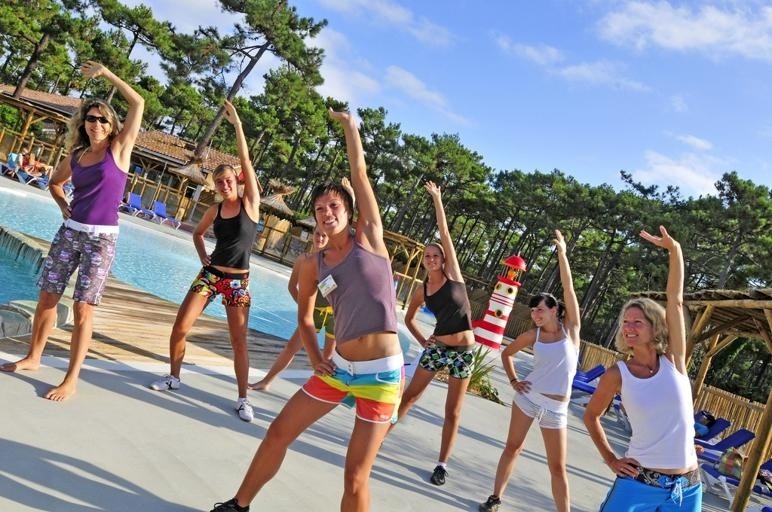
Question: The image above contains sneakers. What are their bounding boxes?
[234,397,254,425]
[207,496,250,512]
[151,374,182,392]
[477,494,503,512]
[429,465,449,488]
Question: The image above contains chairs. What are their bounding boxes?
[570,364,771,512]
[1,153,181,230]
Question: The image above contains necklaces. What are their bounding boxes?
[646,364,657,376]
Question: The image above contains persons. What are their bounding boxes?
[582,223,707,512]
[149,98,263,422]
[384,180,479,487]
[0,61,147,404]
[6,147,29,178]
[694,410,716,438]
[247,175,357,392]
[209,104,407,510]
[477,228,582,511]
[23,152,43,177]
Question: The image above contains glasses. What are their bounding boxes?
[83,115,110,124]
